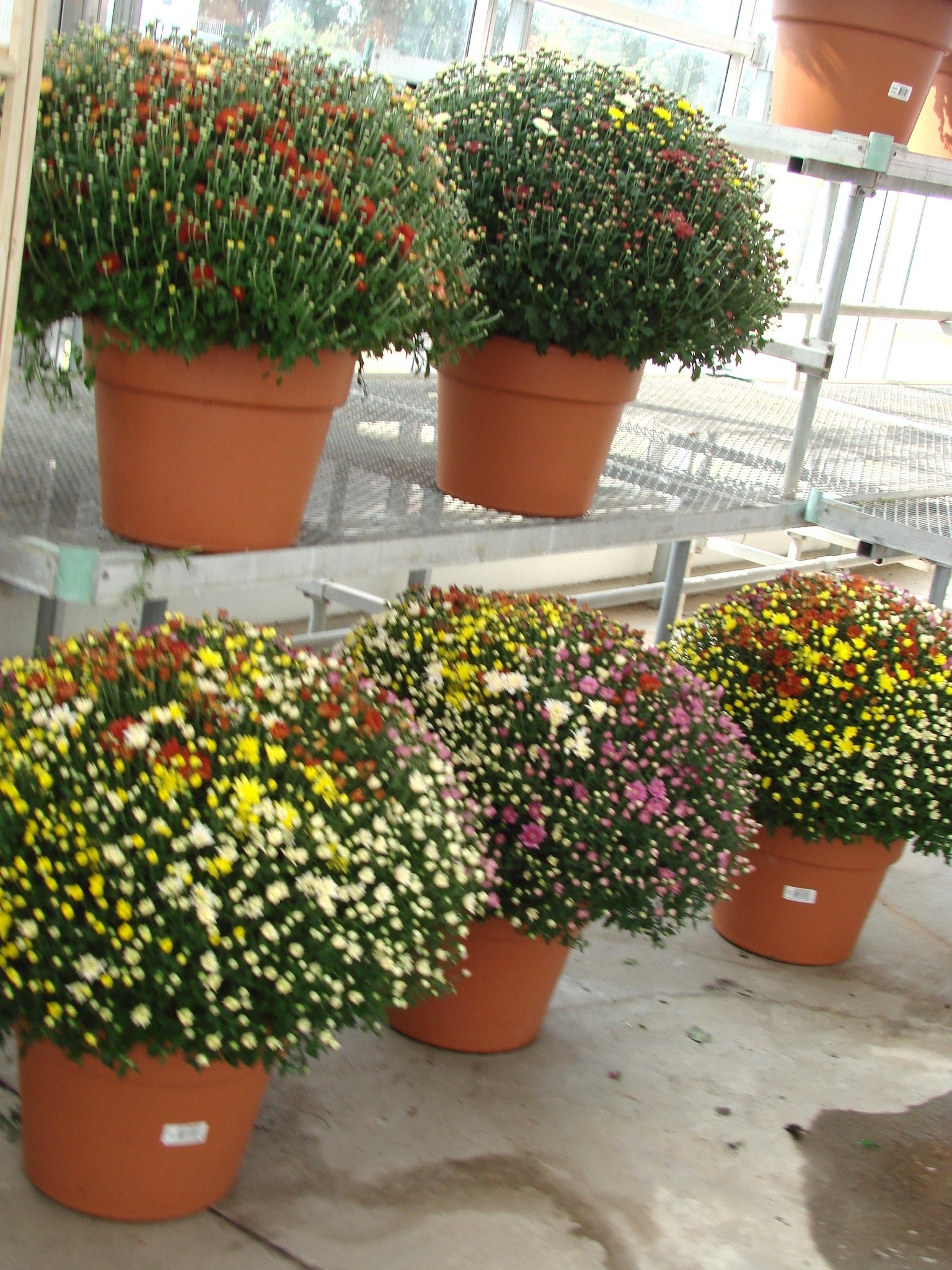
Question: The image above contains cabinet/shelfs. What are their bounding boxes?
[0,1,952,659]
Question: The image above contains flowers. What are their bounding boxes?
[18,21,489,357]
[341,587,756,945]
[0,602,487,1067]
[403,48,791,376]
[665,572,952,867]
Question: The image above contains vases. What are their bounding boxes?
[80,313,358,553]
[432,319,647,520]
[714,806,902,967]
[13,1025,272,1221]
[394,907,594,1053]
[770,0,952,159]
